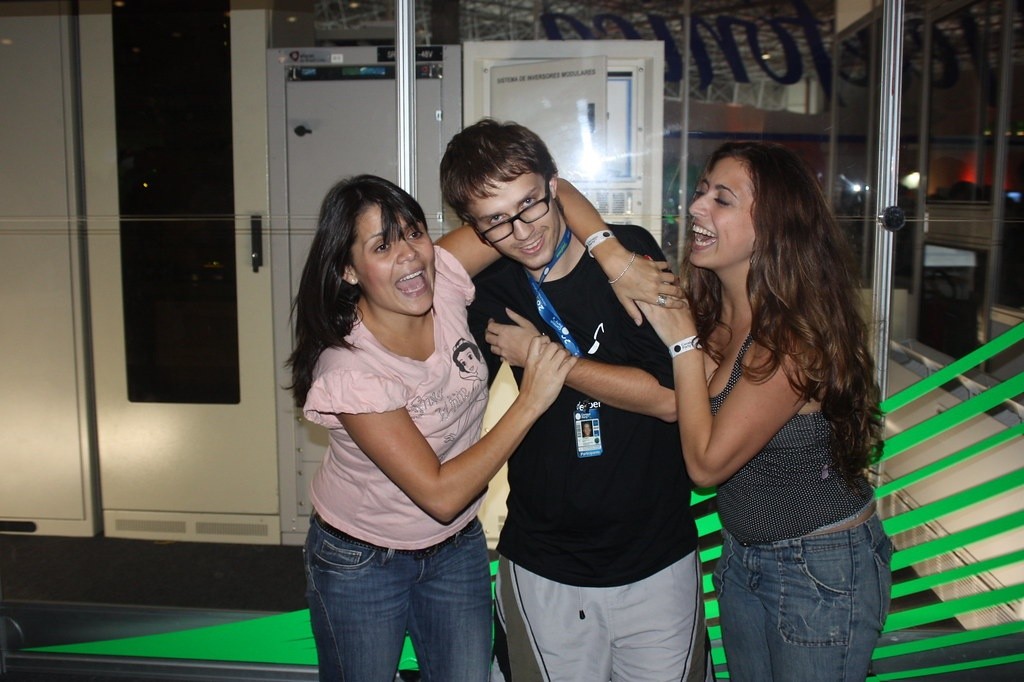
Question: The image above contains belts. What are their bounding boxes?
[312,514,479,559]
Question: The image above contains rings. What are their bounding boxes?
[657,294,667,307]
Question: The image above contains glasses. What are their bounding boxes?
[471,180,551,244]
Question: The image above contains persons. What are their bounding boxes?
[630,138,895,682]
[437,119,704,682]
[291,175,686,682]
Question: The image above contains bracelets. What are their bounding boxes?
[585,230,637,286]
[669,335,703,359]
